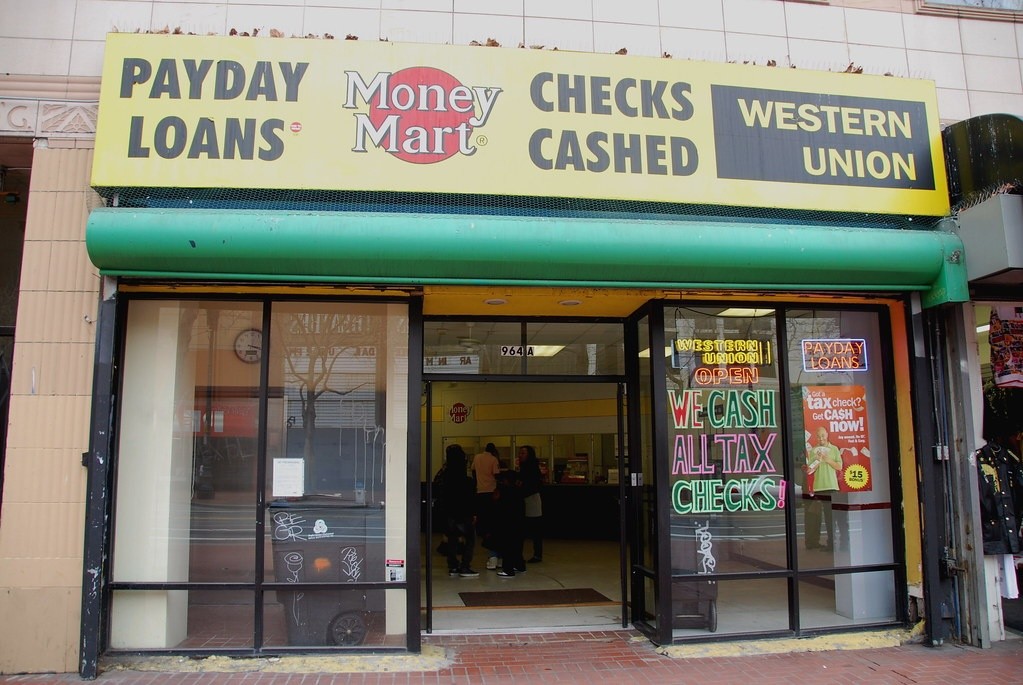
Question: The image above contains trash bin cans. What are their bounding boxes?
[669,502,719,632]
[266,494,385,647]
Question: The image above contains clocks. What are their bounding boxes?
[233,327,261,363]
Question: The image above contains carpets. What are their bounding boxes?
[458,587,614,607]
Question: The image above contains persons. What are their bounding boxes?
[431,443,546,578]
[804,500,850,552]
[808,426,842,495]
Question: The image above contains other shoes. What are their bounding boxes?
[512,567,526,574]
[497,572,514,578]
[497,559,503,567]
[486,557,498,569]
[459,567,480,577]
[448,568,460,576]
[525,557,542,564]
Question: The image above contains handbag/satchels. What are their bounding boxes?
[437,536,465,555]
[523,492,542,517]
